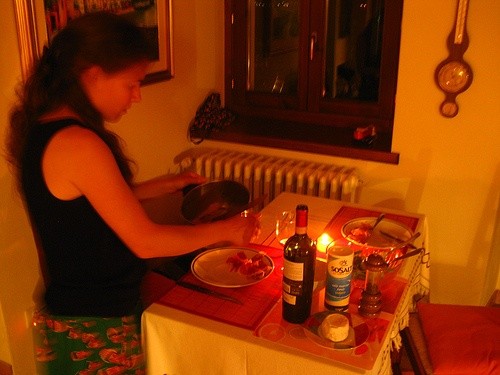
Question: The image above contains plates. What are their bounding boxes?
[340,216,414,249]
[189,247,274,288]
[302,310,370,350]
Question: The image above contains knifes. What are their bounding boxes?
[175,279,243,304]
[378,229,418,250]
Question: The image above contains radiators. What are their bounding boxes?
[178,145,361,209]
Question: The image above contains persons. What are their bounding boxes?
[6,14,260,374]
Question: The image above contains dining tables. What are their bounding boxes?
[142,190,433,375]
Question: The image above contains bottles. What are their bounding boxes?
[282,203,317,323]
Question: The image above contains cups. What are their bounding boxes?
[325,239,354,313]
[276,209,297,248]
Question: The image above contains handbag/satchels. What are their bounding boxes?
[187,93,242,144]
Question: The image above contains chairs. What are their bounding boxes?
[395,287,500,375]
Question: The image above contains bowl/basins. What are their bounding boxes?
[354,248,404,278]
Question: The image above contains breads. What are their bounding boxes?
[322,313,349,342]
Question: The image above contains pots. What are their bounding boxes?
[179,179,251,225]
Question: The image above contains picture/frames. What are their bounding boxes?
[10,0,176,88]
